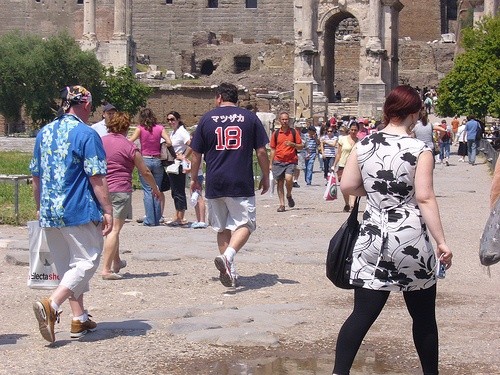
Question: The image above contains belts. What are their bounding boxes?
[143,154,161,159]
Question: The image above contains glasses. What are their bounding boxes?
[166,117,176,122]
[327,130,333,133]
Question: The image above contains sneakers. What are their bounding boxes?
[231,275,240,287]
[215,256,233,288]
[33,300,63,342]
[71,313,97,336]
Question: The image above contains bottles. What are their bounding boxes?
[189,189,199,207]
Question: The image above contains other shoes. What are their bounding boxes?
[458,158,475,166]
[344,205,349,211]
[168,220,185,227]
[112,260,127,272]
[287,194,294,207]
[184,221,197,227]
[293,182,300,187]
[445,161,449,166]
[193,222,208,228]
[138,222,156,226]
[158,216,164,223]
[277,205,285,211]
[101,273,121,280]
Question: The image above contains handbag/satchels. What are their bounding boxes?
[323,172,338,201]
[326,195,361,290]
[480,197,500,266]
[475,128,482,141]
[26,219,72,289]
[441,133,449,142]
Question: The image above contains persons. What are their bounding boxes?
[331,85,453,375]
[29,86,113,342]
[187,125,207,229]
[127,108,172,227]
[90,104,117,136]
[294,84,484,188]
[268,112,303,211]
[478,150,500,266]
[100,112,161,280]
[164,110,193,226]
[332,122,360,212]
[188,82,271,288]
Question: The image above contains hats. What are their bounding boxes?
[103,104,117,113]
